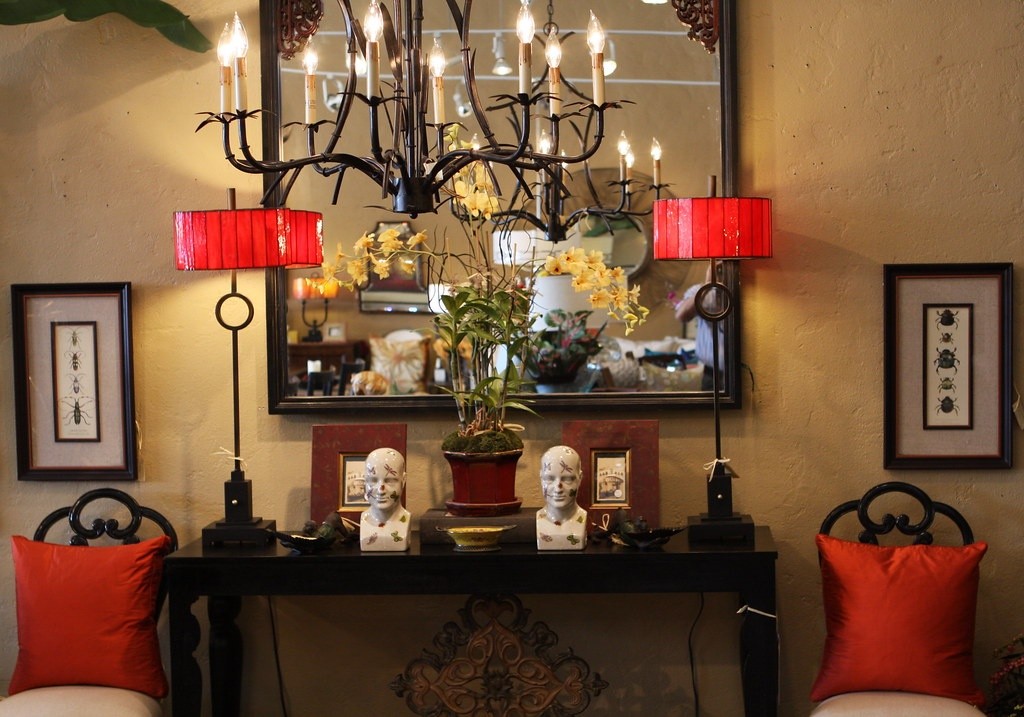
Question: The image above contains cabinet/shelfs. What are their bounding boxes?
[288,340,368,388]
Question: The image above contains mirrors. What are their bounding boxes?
[514,169,691,325]
[260,1,741,416]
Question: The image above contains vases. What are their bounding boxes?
[448,354,464,382]
[534,371,598,394]
[443,453,519,504]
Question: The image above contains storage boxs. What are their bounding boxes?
[419,507,543,546]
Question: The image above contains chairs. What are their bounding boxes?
[591,357,648,392]
[810,482,986,717]
[287,358,366,396]
[0,488,178,717]
[385,329,423,341]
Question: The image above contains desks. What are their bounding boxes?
[165,526,780,716]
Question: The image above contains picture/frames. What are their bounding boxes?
[562,419,660,536]
[322,322,347,344]
[11,282,139,481]
[884,263,1013,471]
[312,423,407,534]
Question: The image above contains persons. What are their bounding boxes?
[600,476,623,499]
[675,258,726,392]
[360,447,411,552]
[347,478,366,502]
[535,446,588,550]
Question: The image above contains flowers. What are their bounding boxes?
[987,633,1024,717]
[305,229,650,452]
[434,337,472,361]
[518,309,608,383]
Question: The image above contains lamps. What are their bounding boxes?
[195,0,624,219]
[173,188,324,553]
[653,175,775,549]
[429,282,474,314]
[525,274,627,332]
[292,277,339,342]
[449,0,680,245]
[322,73,345,112]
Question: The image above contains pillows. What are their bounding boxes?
[370,335,432,395]
[812,533,989,708]
[9,535,170,699]
[614,335,705,392]
